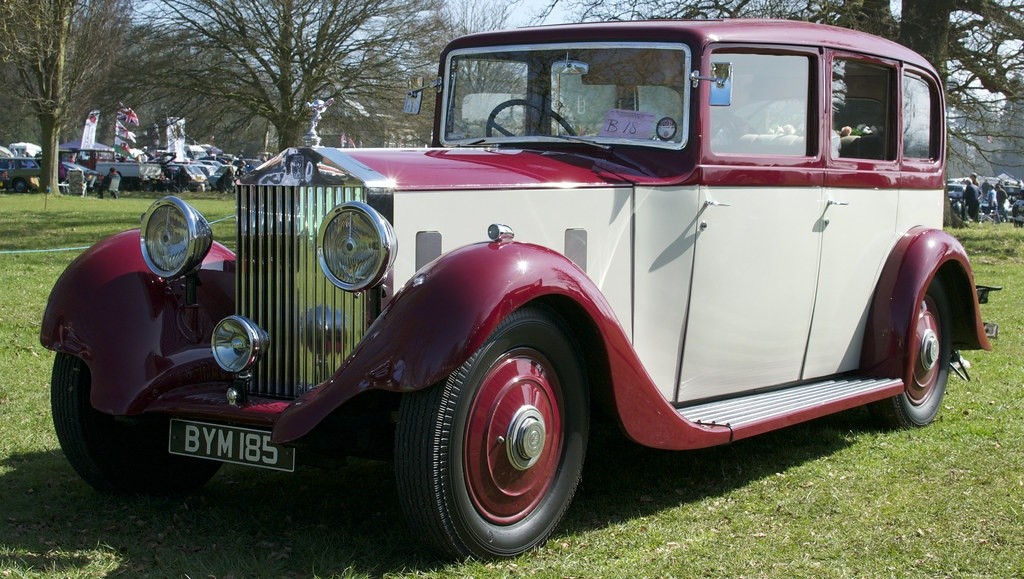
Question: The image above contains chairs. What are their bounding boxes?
[59,168,120,199]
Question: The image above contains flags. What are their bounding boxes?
[114,102,140,159]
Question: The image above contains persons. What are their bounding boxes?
[98,167,123,199]
[965,173,1024,223]
[70,155,75,162]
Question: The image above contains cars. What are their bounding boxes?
[1,137,270,194]
[944,171,1024,229]
[39,16,999,565]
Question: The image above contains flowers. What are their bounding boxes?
[840,123,874,137]
[769,123,796,135]
